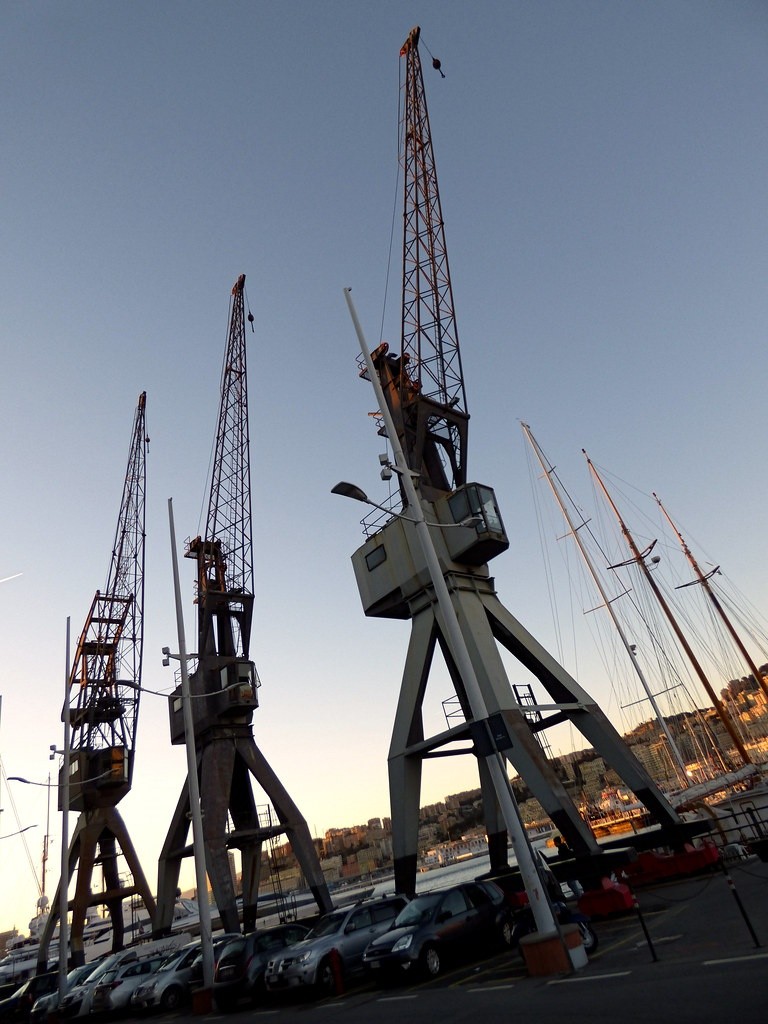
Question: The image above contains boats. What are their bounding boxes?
[0,694,375,984]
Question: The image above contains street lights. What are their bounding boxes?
[114,495,253,1012]
[331,287,589,978]
[5,614,118,1010]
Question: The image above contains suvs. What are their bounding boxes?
[266,892,429,1005]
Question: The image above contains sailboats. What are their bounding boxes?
[515,416,768,847]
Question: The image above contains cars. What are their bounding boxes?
[364,878,514,979]
[0,876,311,1024]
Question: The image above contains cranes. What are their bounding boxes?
[61,391,152,750]
[183,274,255,667]
[355,26,471,510]
